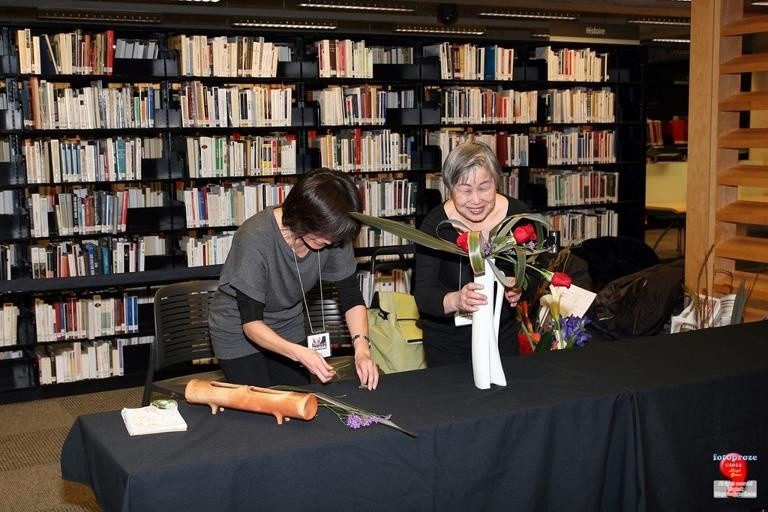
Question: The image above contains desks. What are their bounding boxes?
[59,316,768,511]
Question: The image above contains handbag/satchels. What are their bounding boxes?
[594,263,681,336]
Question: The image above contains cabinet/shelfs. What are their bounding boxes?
[0,25,649,404]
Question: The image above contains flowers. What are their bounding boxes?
[513,293,593,354]
[344,209,573,309]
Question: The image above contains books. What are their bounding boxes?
[3,26,620,390]
[120,405,188,437]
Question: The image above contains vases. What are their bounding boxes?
[471,258,511,389]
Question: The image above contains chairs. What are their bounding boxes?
[381,291,424,373]
[140,281,228,406]
[569,236,660,296]
[302,281,359,383]
